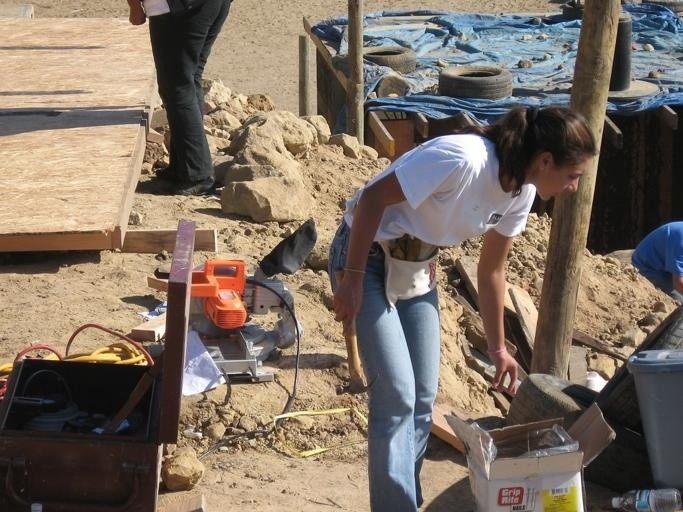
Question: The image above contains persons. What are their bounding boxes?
[127,0,233,196]
[629,221,683,305]
[327,103,596,512]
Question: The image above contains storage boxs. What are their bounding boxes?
[444,399,617,512]
[0,218,202,512]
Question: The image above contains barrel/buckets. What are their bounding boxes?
[609,16,633,92]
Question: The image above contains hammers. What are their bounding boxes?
[334,271,378,395]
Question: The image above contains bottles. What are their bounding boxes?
[610,488,681,512]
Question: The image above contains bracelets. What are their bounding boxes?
[486,346,507,355]
[343,268,366,274]
